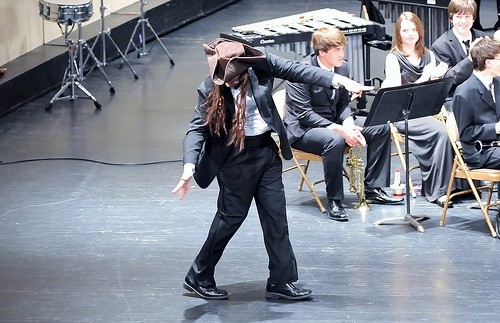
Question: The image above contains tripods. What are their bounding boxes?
[365,76,457,232]
[45,0,175,109]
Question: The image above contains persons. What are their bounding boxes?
[282,27,404,221]
[171,37,375,301]
[430,0,491,153]
[385,12,482,208]
[451,38,500,239]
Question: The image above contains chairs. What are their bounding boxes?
[269,88,500,238]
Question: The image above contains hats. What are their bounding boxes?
[202,37,267,85]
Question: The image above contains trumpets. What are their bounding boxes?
[346,131,372,210]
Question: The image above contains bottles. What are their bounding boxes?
[394,169,400,186]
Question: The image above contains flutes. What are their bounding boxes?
[474,140,500,153]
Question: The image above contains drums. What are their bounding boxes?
[38,0,94,25]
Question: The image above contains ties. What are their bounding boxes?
[462,39,470,56]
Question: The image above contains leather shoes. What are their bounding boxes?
[365,187,404,204]
[476,190,483,194]
[265,278,312,299]
[437,193,453,206]
[326,199,348,219]
[183,276,228,298]
